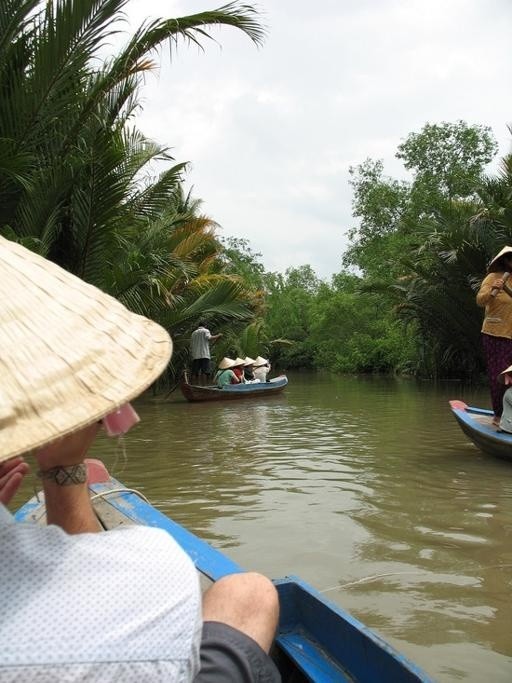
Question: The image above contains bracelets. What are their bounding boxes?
[37,463,87,486]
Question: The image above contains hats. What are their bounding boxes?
[253,356,267,366]
[218,356,235,369]
[0,227,174,466]
[235,358,245,366]
[243,356,255,366]
[487,246,512,275]
[497,365,512,385]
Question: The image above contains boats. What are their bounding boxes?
[10,451,440,683]
[448,399,512,462]
[180,368,290,403]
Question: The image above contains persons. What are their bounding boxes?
[499,365,512,434]
[0,233,283,683]
[189,321,223,389]
[476,246,512,425]
[213,355,272,389]
